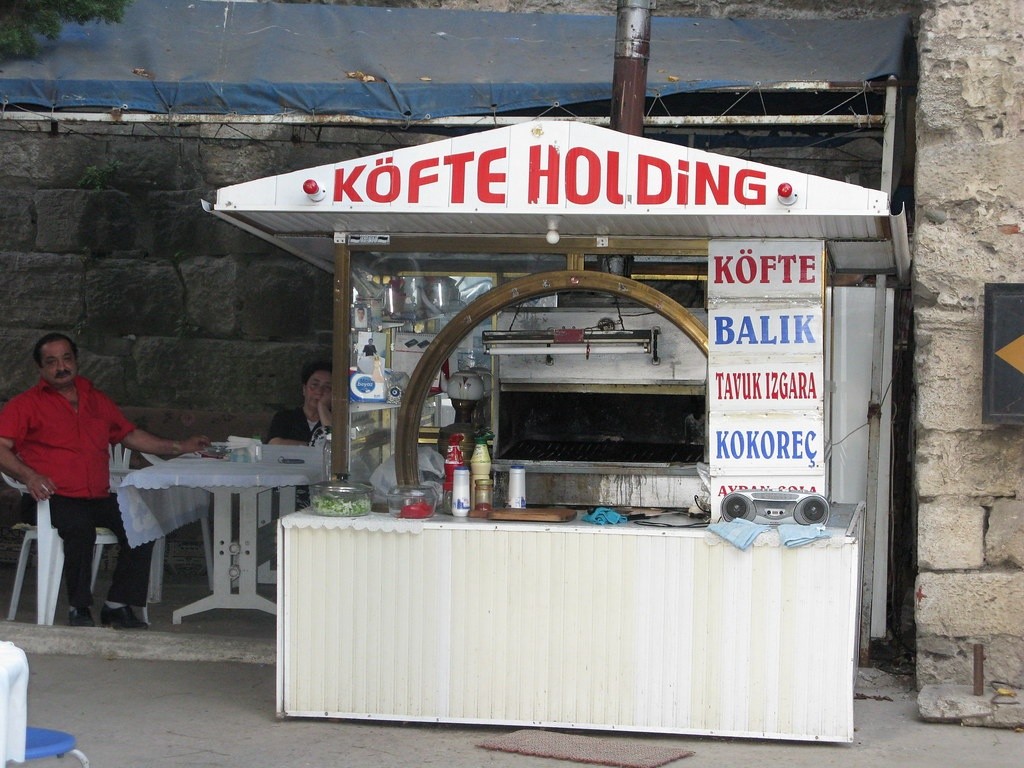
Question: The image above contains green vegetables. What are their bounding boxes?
[312,495,367,515]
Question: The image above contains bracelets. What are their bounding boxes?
[173,440,181,454]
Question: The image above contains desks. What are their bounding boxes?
[125,444,327,624]
[273,503,861,745]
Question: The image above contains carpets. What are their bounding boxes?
[476,728,695,768]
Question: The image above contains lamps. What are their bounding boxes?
[481,254,652,355]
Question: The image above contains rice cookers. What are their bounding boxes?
[425,276,461,315]
[392,276,425,314]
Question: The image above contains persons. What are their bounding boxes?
[266,360,333,513]
[355,308,367,328]
[359,338,377,360]
[0,333,213,628]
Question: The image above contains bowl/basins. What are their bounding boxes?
[387,485,439,518]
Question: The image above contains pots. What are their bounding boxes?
[308,472,376,516]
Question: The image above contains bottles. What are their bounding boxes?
[470,434,495,510]
[452,466,470,516]
[444,434,464,514]
[475,479,492,512]
[509,466,526,508]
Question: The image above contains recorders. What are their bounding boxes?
[720,489,830,528]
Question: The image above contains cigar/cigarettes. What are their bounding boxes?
[42,484,50,493]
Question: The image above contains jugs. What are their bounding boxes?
[471,363,492,396]
[440,365,492,401]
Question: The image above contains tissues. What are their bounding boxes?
[225,435,263,464]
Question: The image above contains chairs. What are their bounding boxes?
[0,442,164,623]
[0,641,88,768]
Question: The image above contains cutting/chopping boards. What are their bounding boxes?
[470,508,577,522]
